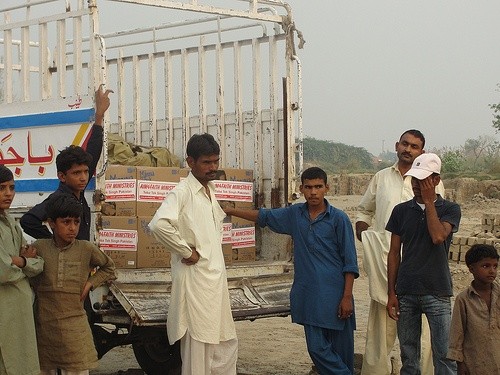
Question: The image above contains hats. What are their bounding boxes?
[402,153,441,181]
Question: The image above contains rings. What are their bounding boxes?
[24,245,28,248]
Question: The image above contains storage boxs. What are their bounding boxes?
[98,162,257,269]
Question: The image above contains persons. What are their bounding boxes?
[355,130,441,375]
[20,84,109,332]
[225,168,359,375]
[147,132,239,375]
[385,153,461,375]
[30,194,116,375]
[446,243,500,375]
[0,164,45,374]
